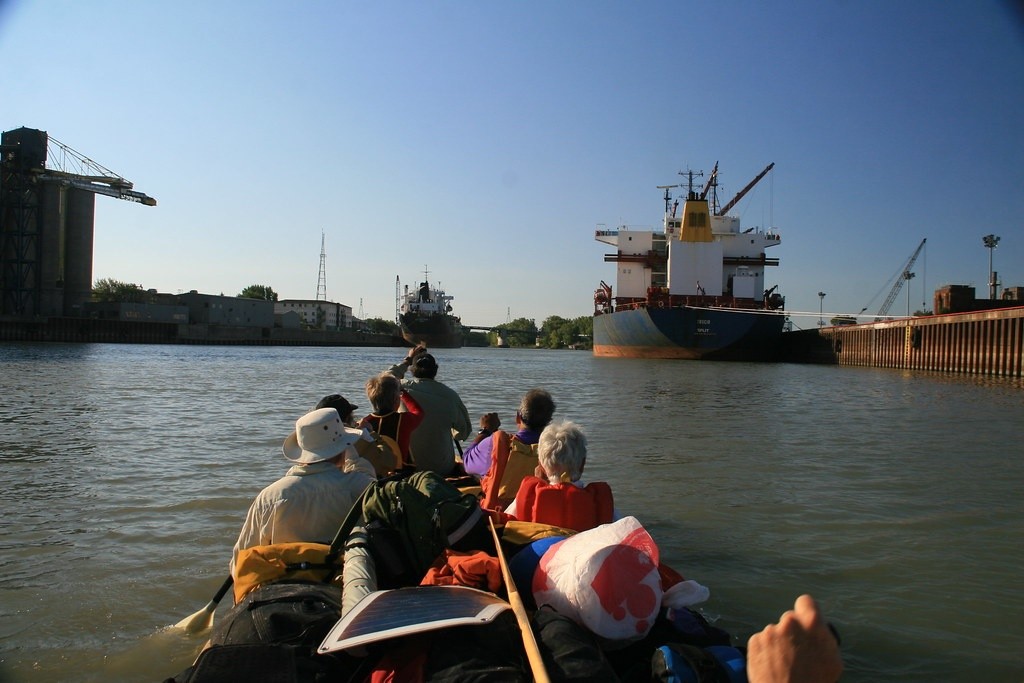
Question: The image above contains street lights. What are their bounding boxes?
[902,271,915,317]
[818,291,826,327]
[981,233,1001,300]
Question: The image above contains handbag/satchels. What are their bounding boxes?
[650,642,748,683]
[161,643,298,683]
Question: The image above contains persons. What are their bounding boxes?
[358,368,424,482]
[228,407,378,580]
[315,395,396,477]
[464,387,558,512]
[509,416,622,532]
[386,345,471,475]
[747,593,844,683]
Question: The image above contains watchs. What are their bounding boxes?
[476,427,492,436]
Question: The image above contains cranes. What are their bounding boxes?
[2,126,157,320]
[854,236,929,324]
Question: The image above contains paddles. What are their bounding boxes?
[165,575,233,640]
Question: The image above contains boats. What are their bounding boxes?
[197,464,758,683]
[401,265,469,348]
[586,155,787,360]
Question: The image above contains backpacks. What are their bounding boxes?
[362,469,497,591]
[530,604,619,683]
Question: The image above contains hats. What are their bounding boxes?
[316,394,358,415]
[282,408,363,463]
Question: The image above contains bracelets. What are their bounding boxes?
[403,356,413,366]
